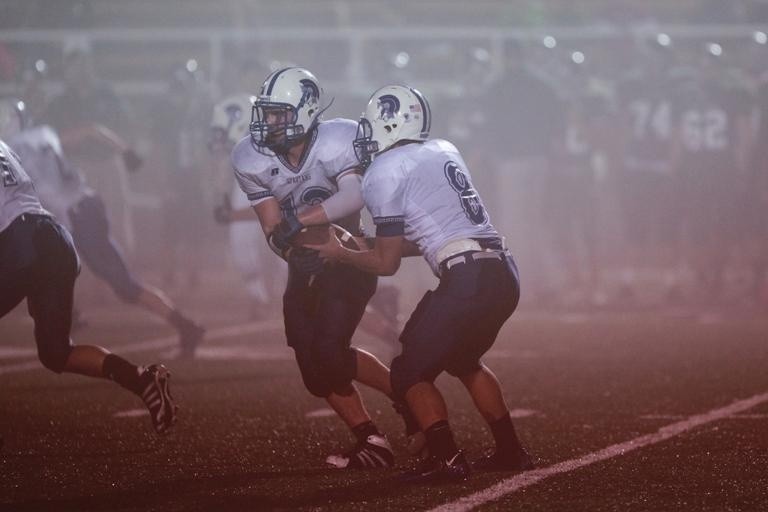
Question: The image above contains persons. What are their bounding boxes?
[289,84,534,479]
[0,96,207,363]
[0,138,181,440]
[231,65,427,472]
[529,31,765,304]
[207,87,418,351]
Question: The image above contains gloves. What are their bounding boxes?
[275,217,303,241]
[289,249,323,277]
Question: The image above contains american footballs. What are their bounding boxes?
[285,222,361,263]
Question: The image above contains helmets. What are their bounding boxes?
[353,85,431,166]
[249,67,323,148]
[207,94,253,151]
[1,97,25,140]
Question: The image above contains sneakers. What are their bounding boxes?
[140,363,179,434]
[396,398,421,436]
[402,452,469,484]
[327,435,396,473]
[178,326,204,360]
[471,447,533,471]
